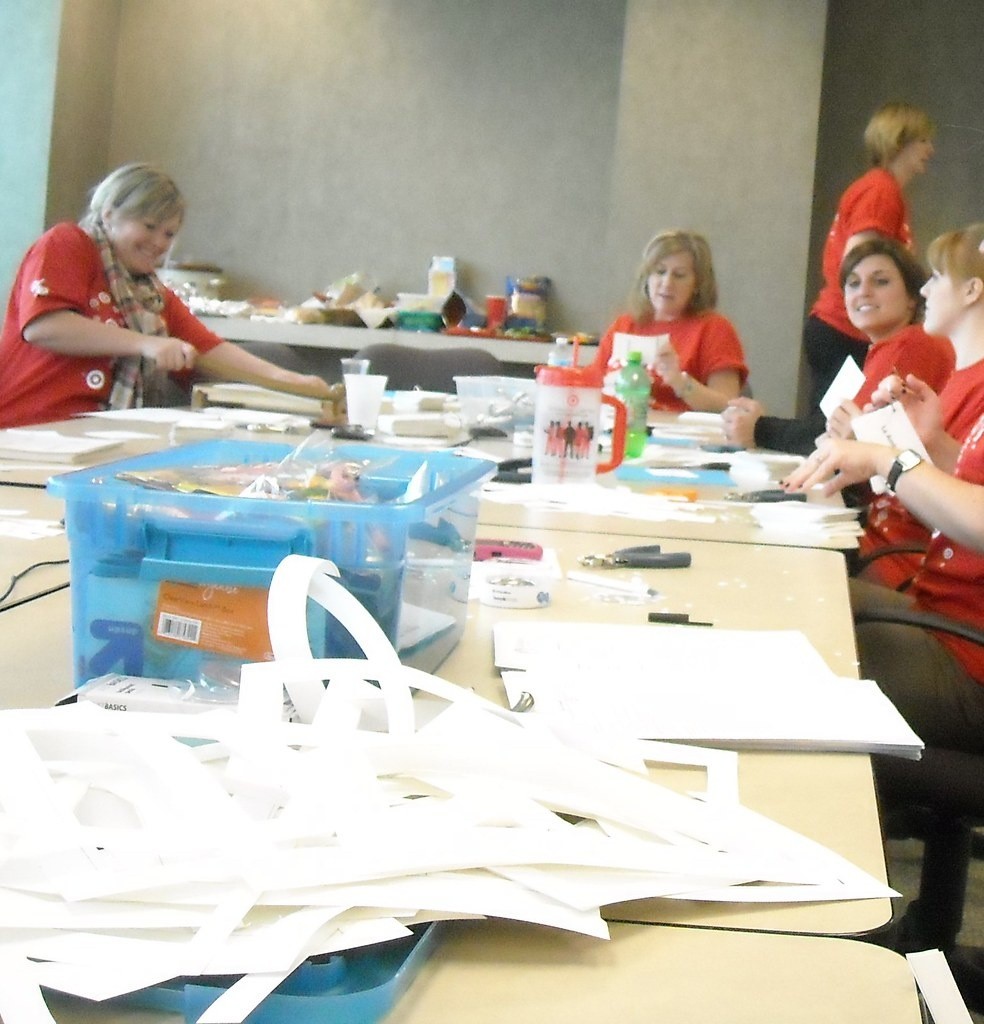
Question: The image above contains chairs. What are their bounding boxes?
[143,341,306,409]
[846,541,984,1015]
[355,345,504,394]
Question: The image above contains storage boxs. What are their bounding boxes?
[45,436,497,701]
[451,373,536,442]
[54,673,303,751]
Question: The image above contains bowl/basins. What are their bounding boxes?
[400,311,441,333]
[399,291,448,310]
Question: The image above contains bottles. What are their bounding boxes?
[547,337,573,369]
[428,253,457,299]
[615,351,650,459]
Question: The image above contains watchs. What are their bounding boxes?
[885,448,927,493]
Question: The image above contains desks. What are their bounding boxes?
[0,404,860,564]
[199,315,598,366]
[0,485,896,941]
[0,918,931,1023]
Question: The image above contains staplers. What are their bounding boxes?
[492,459,533,483]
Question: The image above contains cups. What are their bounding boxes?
[530,314,633,501]
[485,295,508,329]
[344,373,388,427]
[341,357,370,374]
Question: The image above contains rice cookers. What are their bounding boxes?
[155,262,225,302]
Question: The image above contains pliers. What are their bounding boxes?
[581,545,692,571]
[725,488,807,504]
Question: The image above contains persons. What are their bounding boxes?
[721,239,956,457]
[803,102,933,438]
[778,412,984,755]
[585,229,748,413]
[0,161,330,429]
